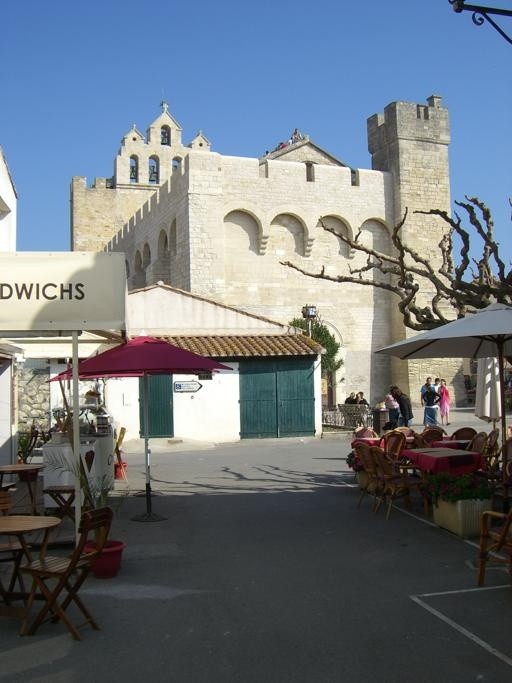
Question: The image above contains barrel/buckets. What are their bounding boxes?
[114,462,128,480]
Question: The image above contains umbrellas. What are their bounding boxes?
[475,356,502,431]
[46,337,233,523]
[373,303,512,482]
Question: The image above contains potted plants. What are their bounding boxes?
[52,454,127,580]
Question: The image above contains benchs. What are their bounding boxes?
[338,404,372,426]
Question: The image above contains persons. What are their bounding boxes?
[385,377,449,430]
[263,128,303,157]
[345,392,369,409]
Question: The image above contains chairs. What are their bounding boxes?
[345,425,512,588]
[115,426,130,481]
[0,462,114,641]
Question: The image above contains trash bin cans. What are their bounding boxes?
[372,409,390,436]
